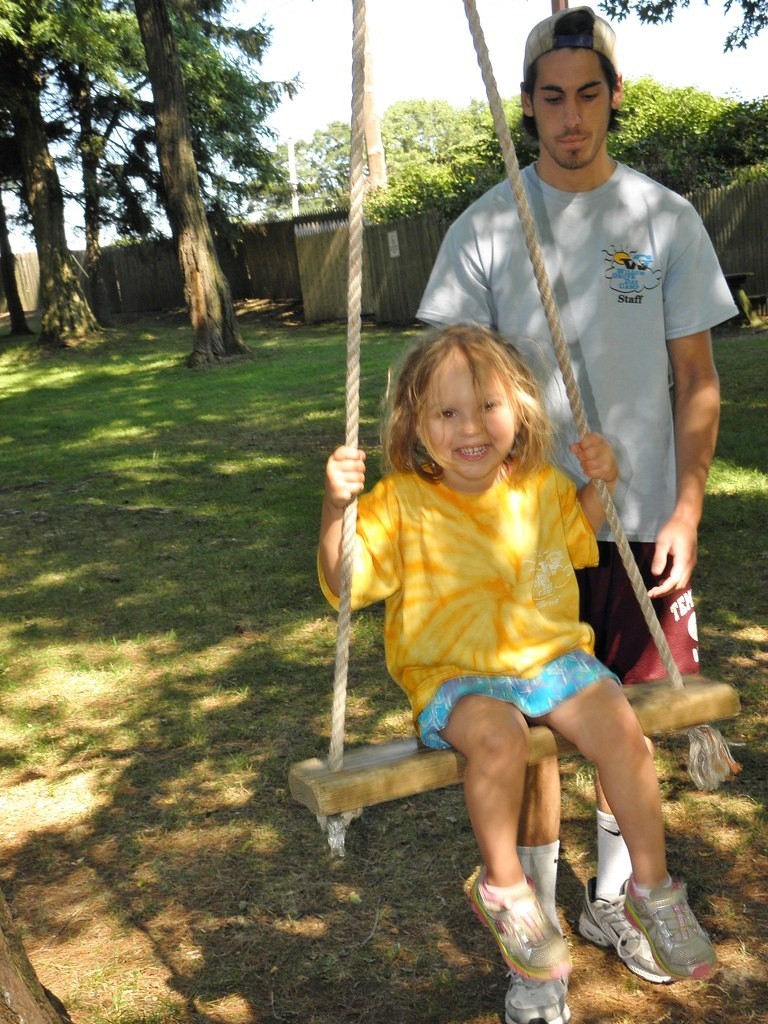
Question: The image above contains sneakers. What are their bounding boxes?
[578,876,677,984]
[504,969,571,1024]
[469,864,573,984]
[624,874,718,980]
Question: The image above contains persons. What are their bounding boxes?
[415,7,740,1024]
[319,323,717,981]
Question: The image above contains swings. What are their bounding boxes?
[286,0,743,818]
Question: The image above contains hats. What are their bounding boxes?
[523,6,618,135]
